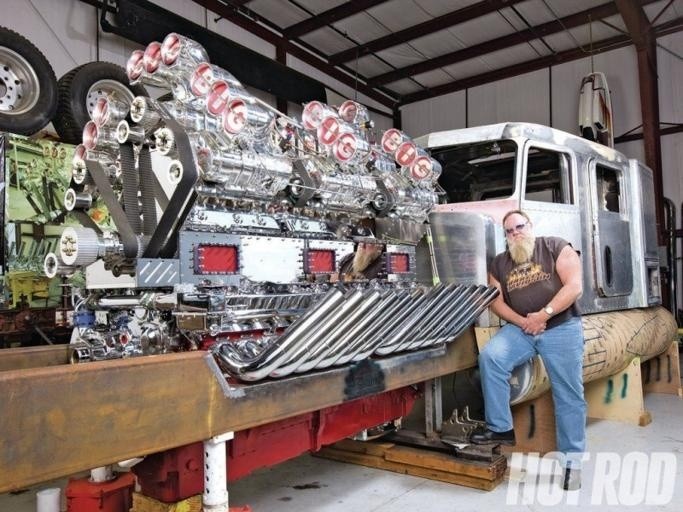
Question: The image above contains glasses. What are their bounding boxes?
[505,220,529,234]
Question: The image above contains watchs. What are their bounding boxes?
[544,306,558,317]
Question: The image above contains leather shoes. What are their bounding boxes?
[559,465,582,490]
[471,426,517,446]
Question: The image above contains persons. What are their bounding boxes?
[469,209,586,491]
